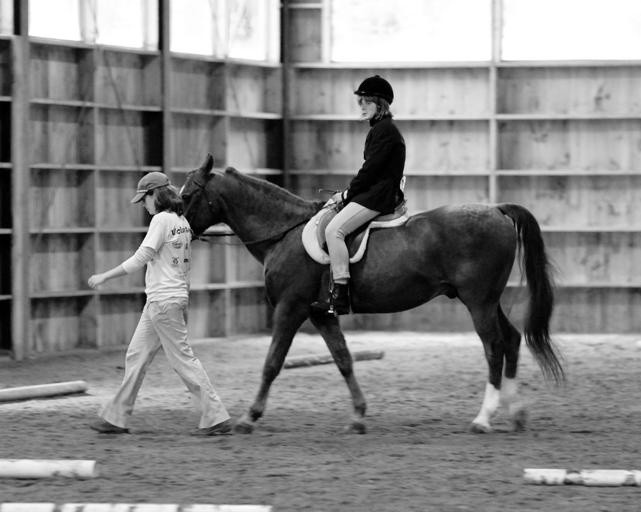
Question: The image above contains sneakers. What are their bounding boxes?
[190,419,232,435]
[90,419,129,433]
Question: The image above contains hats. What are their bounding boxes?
[131,172,170,204]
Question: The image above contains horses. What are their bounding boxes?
[175,152,569,435]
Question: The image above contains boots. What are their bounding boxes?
[312,284,351,312]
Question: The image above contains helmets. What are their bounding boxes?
[353,76,394,104]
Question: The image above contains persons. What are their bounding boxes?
[311,74,407,316]
[87,171,235,438]
